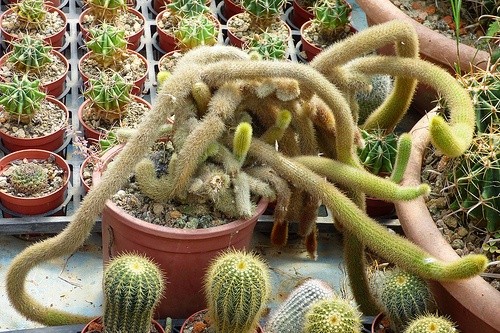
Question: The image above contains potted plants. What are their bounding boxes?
[0,0,500,333]
